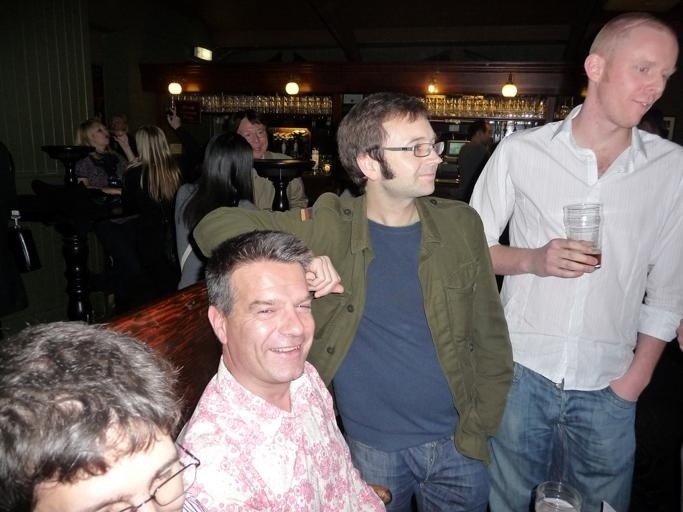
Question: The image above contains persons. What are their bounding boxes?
[73,118,136,195]
[468,15,683,511]
[0,322,200,512]
[224,110,309,210]
[176,230,386,512]
[175,133,258,290]
[106,111,138,160]
[121,107,203,252]
[460,120,496,201]
[193,92,512,511]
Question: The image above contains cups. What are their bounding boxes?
[534,481,582,512]
[562,206,604,269]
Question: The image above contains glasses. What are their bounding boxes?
[243,129,267,139]
[383,141,446,157]
[119,434,200,512]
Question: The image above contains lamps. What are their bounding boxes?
[502,71,517,97]
[427,71,439,94]
[168,75,182,95]
[285,73,300,95]
